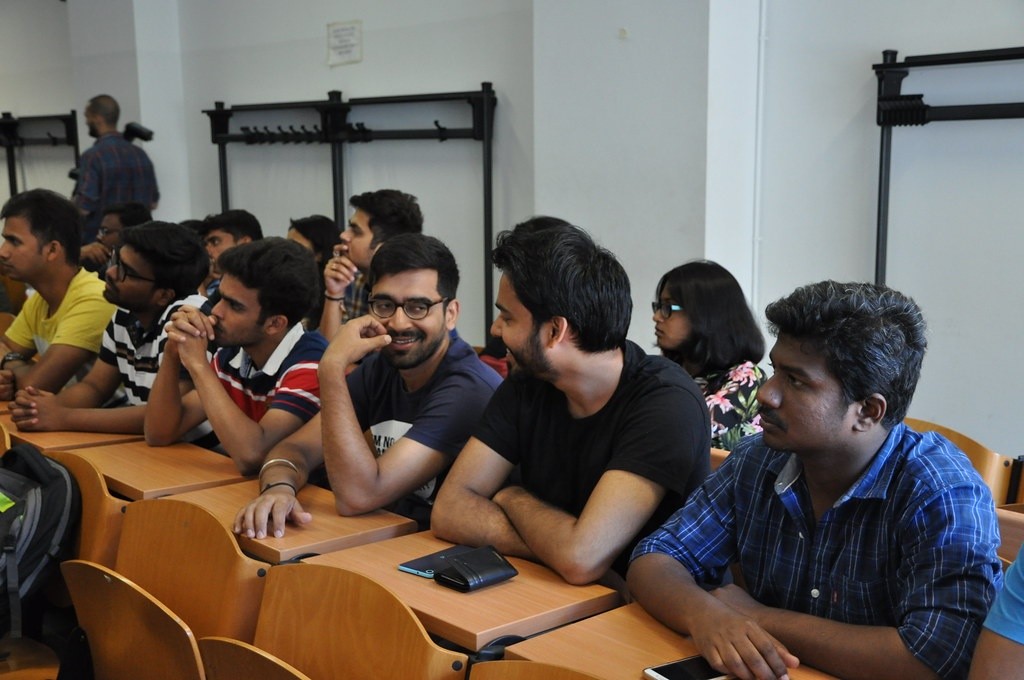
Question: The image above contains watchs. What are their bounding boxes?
[0,351,28,370]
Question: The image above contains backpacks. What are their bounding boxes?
[0,439,82,652]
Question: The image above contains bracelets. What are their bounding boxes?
[258,458,298,481]
[260,482,297,497]
[324,290,346,301]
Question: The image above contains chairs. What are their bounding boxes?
[0,419,1024,680]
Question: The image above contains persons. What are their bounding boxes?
[233,232,506,538]
[650,259,770,451]
[967,542,1024,680]
[70,94,161,243]
[627,280,1004,680]
[0,189,426,477]
[430,214,712,585]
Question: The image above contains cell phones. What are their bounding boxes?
[399,545,479,579]
[643,654,738,680]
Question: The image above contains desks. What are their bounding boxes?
[59,440,254,500]
[157,479,417,564]
[0,411,146,454]
[504,602,840,680]
[299,529,618,652]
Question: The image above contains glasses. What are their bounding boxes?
[97,228,127,236]
[652,299,684,320]
[366,298,449,320]
[110,244,163,285]
